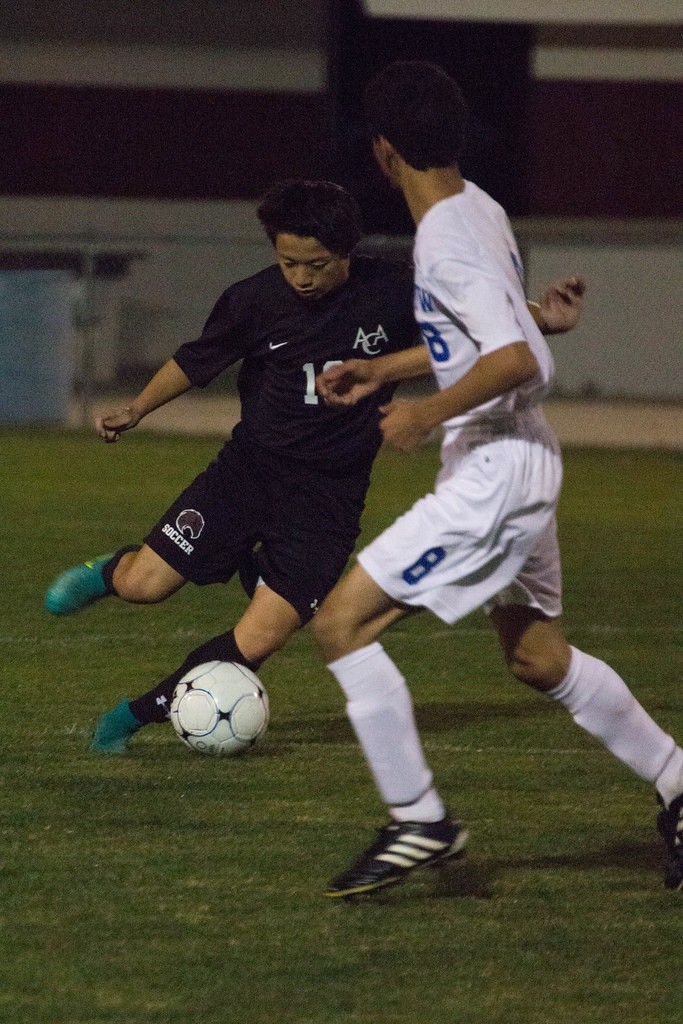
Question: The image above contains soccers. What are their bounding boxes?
[168,658,272,758]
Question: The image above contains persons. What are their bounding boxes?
[42,59,683,902]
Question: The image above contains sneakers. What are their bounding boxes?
[91,703,142,755]
[324,814,468,897]
[44,553,117,616]
[658,795,683,890]
